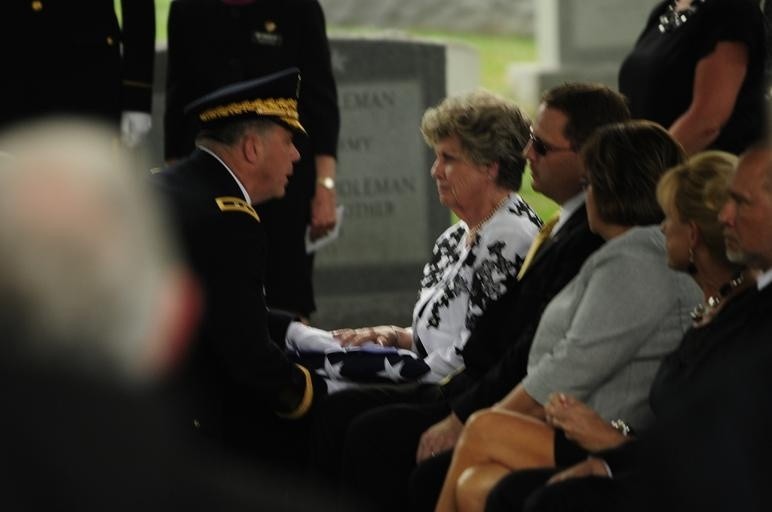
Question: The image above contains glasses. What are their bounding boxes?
[530,127,574,156]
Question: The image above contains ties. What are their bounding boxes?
[515,216,559,280]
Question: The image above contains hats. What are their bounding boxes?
[183,65,307,137]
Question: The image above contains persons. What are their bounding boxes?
[1,3,772,512]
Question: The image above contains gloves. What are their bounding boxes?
[120,112,152,148]
